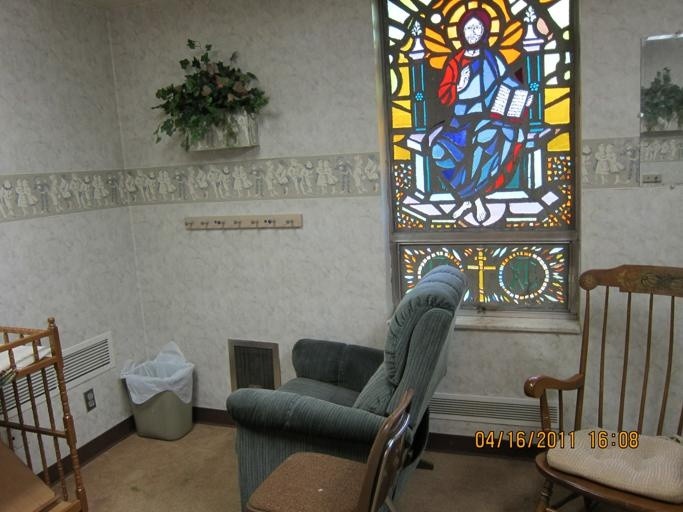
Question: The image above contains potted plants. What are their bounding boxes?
[641,69,683,164]
[154,38,269,152]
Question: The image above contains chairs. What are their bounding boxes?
[225,266,468,511]
[524,265,683,512]
[245,387,414,512]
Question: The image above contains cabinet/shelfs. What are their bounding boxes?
[0,317,88,512]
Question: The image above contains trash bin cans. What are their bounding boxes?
[123,360,195,441]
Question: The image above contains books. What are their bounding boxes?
[487,81,532,126]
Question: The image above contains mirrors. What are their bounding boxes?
[641,35,683,185]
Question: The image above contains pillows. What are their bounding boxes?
[355,357,393,411]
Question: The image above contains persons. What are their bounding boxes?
[578,133,683,189]
[0,153,381,222]
[421,8,543,230]
